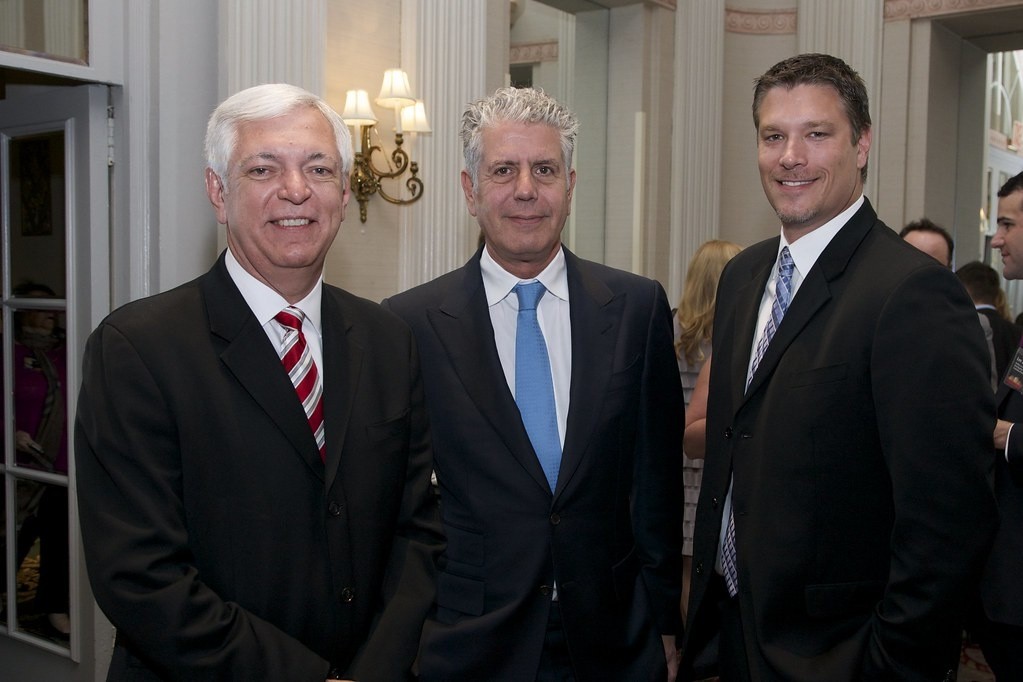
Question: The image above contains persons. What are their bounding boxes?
[672,240,741,620]
[899,167,1023,681]
[381,88,686,682]
[675,54,999,681]
[75,84,447,682]
[0,285,72,641]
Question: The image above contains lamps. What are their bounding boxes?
[341,68,434,221]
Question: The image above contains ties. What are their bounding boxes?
[274,304,327,466]
[720,245,795,601]
[510,281,562,496]
